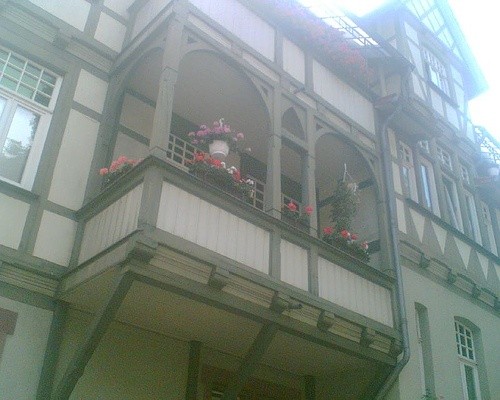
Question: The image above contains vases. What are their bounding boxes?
[207,139,231,159]
[281,214,310,232]
[102,164,133,189]
[189,167,250,202]
[325,237,368,262]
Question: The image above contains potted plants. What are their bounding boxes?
[325,178,361,232]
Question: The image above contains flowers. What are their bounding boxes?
[184,152,255,195]
[99,155,145,175]
[281,201,314,219]
[187,119,251,153]
[323,225,369,254]
